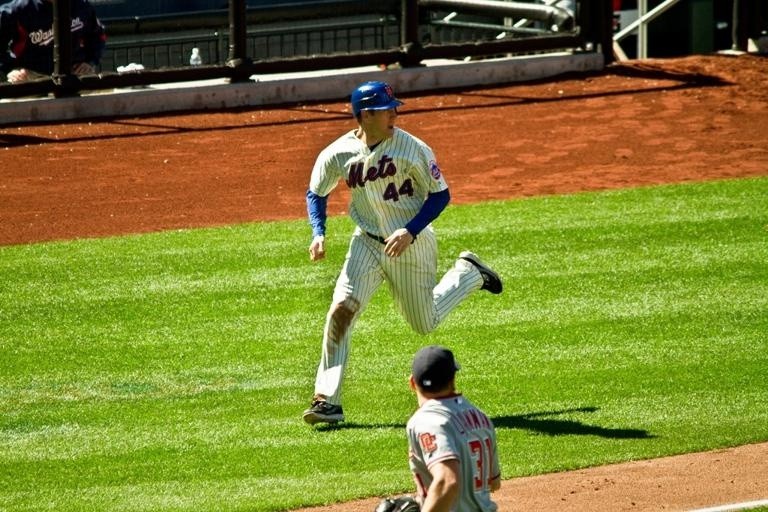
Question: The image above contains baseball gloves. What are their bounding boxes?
[375,496,419,512]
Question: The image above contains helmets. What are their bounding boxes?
[352,80,405,115]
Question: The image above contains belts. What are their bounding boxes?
[367,232,387,245]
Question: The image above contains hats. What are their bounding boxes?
[412,346,461,392]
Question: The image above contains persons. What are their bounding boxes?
[405,346,501,512]
[0,0,107,84]
[303,80,501,428]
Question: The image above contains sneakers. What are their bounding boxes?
[459,251,502,294]
[303,401,344,424]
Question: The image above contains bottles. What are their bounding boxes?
[189,47,202,66]
